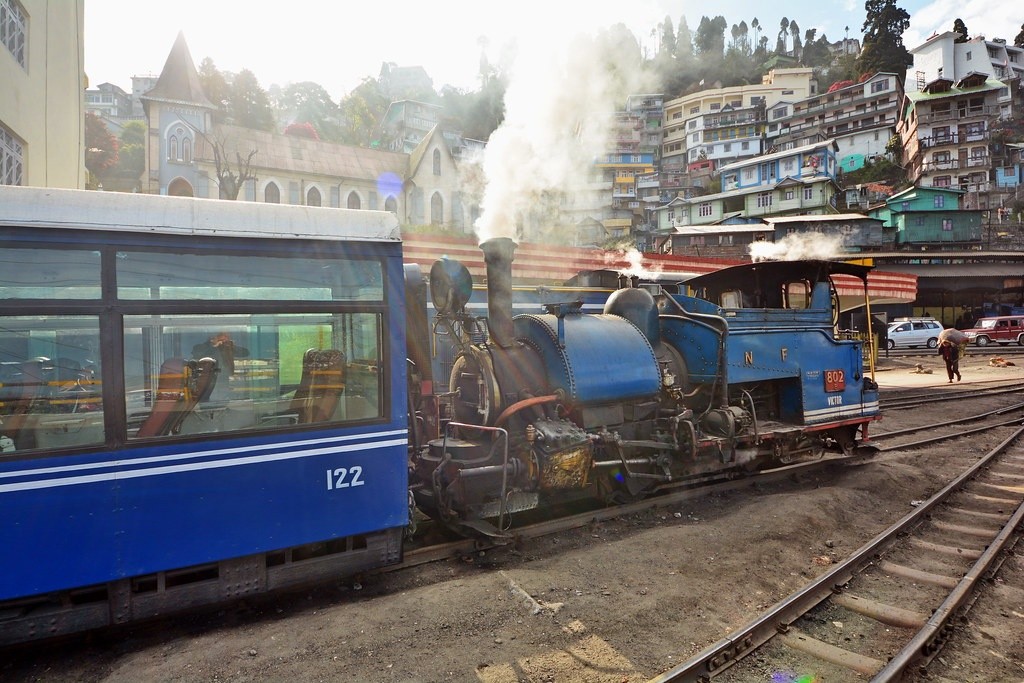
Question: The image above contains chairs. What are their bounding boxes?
[0,348,349,455]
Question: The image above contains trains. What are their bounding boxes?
[0,185,886,651]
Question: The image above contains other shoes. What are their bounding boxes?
[948,380,953,383]
[957,375,961,381]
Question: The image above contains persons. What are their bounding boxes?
[191,333,250,410]
[938,336,961,383]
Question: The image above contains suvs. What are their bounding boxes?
[960,315,1024,347]
[884,316,944,350]
